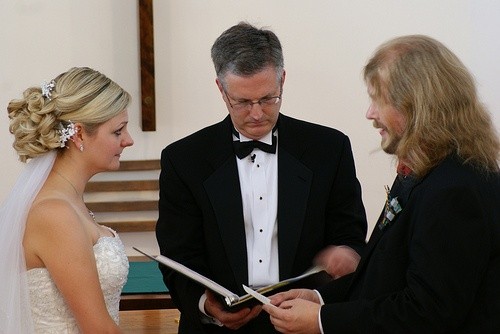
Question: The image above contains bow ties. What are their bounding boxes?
[232,135,278,160]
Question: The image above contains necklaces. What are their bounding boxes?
[51,169,96,222]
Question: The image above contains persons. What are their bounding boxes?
[154,21,368,334]
[0,66,135,334]
[261,35,500,334]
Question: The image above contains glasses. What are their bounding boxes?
[221,73,283,110]
